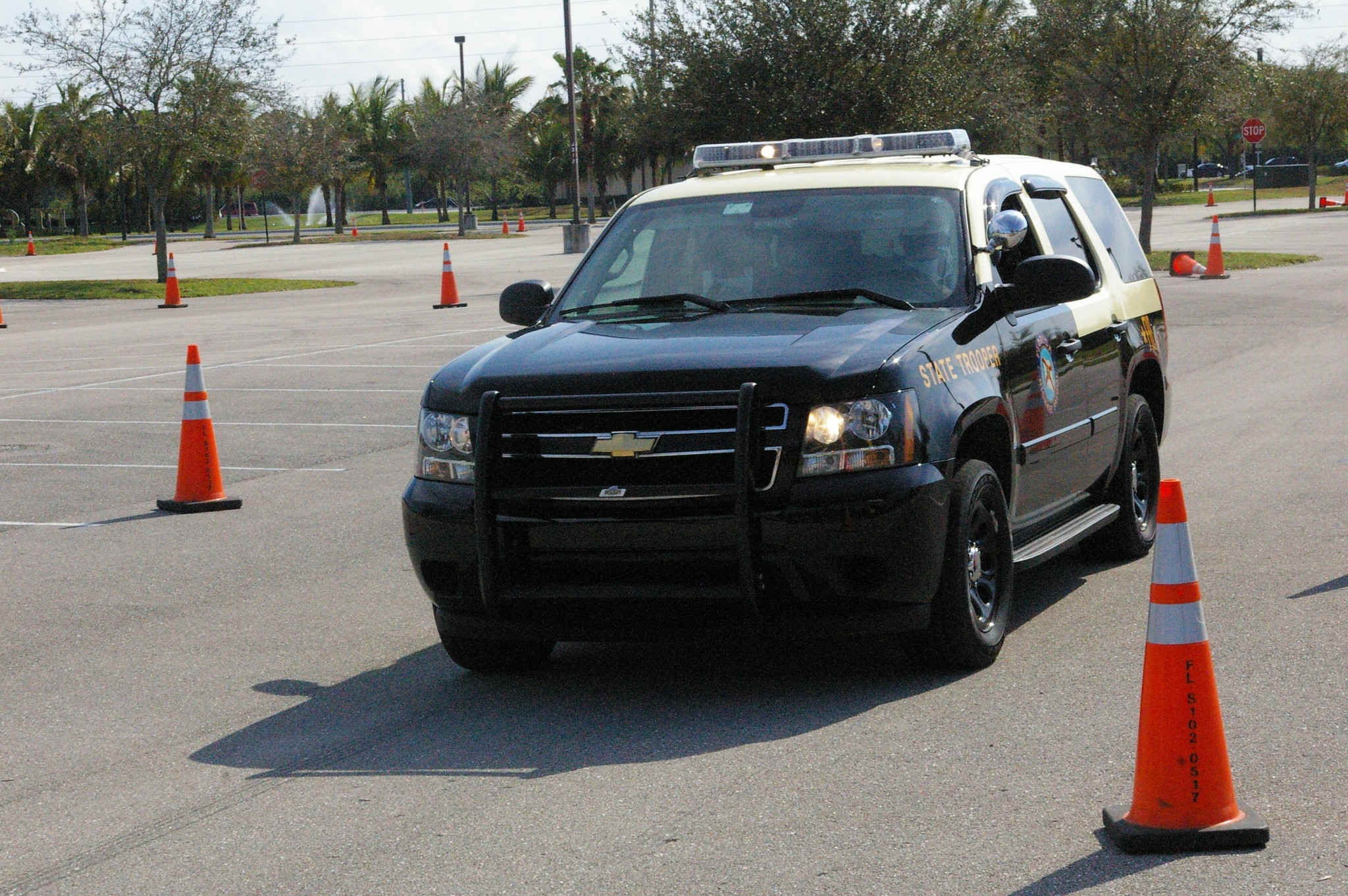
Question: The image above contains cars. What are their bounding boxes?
[1193,162,1227,179]
[1181,168,1195,177]
[1246,156,1299,178]
[1334,158,1348,168]
[413,195,459,209]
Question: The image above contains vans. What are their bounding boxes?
[220,201,258,217]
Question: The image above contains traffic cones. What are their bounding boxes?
[1168,250,1209,279]
[1341,179,1348,207]
[157,253,191,308]
[1319,196,1343,208]
[432,242,467,309]
[516,209,528,232]
[26,230,37,256]
[1097,478,1271,857]
[1200,214,1232,280]
[154,344,249,515]
[1205,180,1218,206]
[0,304,8,328]
[500,213,509,235]
[350,218,359,237]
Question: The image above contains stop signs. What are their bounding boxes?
[253,170,271,189]
[1242,118,1268,143]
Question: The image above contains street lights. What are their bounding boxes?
[454,35,479,230]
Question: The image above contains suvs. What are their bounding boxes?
[400,128,1174,672]
